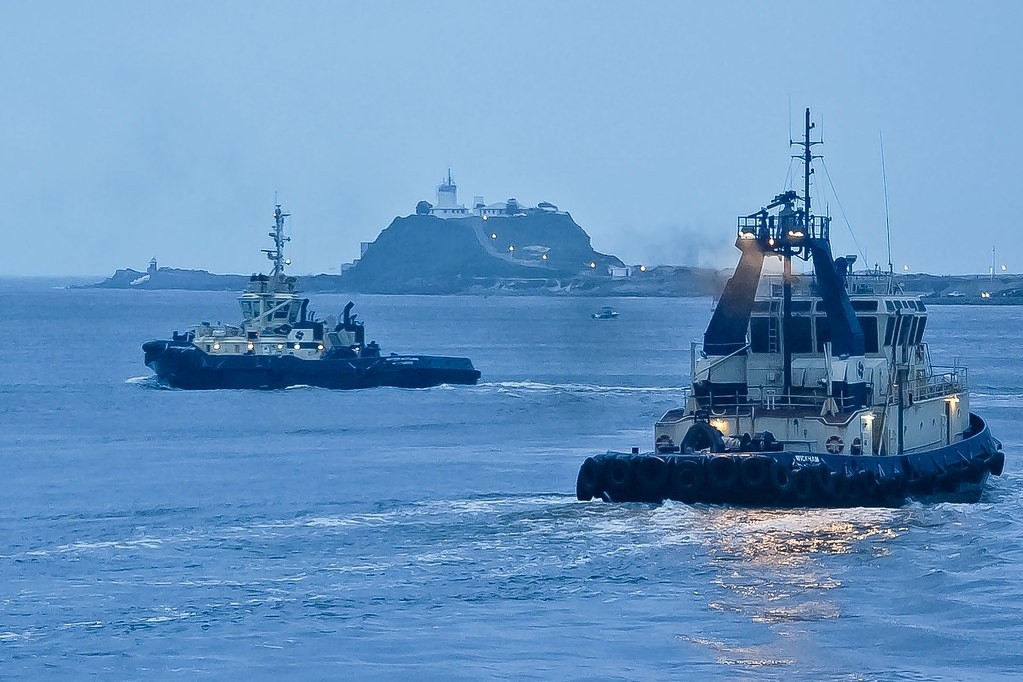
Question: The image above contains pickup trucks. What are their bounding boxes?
[947,291,965,298]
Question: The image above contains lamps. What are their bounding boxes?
[739,227,754,238]
[285,258,291,266]
[317,345,325,350]
[789,224,805,237]
[213,344,221,350]
[248,343,255,349]
[278,344,284,349]
[294,344,300,350]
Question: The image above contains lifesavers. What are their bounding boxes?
[835,469,875,501]
[825,435,844,454]
[575,455,829,505]
[990,451,1005,476]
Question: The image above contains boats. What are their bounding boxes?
[142,191,483,390]
[590,306,620,320]
[576,97,1005,505]
